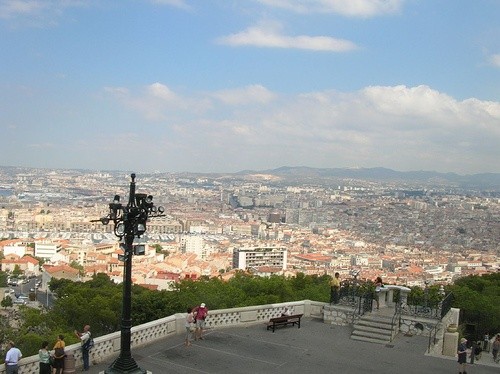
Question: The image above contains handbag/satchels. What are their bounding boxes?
[55,341,65,357]
[49,355,54,365]
[189,323,196,332]
[193,317,197,324]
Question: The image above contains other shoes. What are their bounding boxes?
[459,371,467,374]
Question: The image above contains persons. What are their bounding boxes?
[281,306,291,327]
[75,325,92,372]
[330,272,342,304]
[373,277,383,309]
[39,341,52,374]
[185,303,208,347]
[52,335,66,374]
[457,338,468,374]
[467,332,500,364]
[5,341,23,374]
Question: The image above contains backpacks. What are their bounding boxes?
[83,334,94,351]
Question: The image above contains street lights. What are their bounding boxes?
[89,172,166,374]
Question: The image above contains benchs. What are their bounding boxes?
[267,314,303,332]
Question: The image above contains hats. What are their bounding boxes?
[461,337,467,343]
[200,303,205,308]
